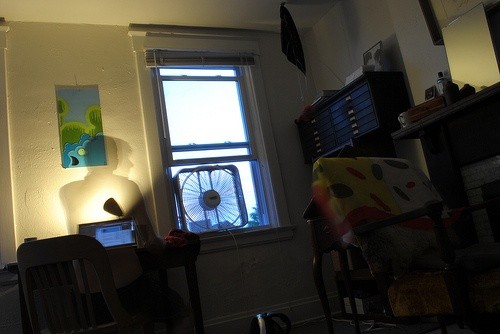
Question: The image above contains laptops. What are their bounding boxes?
[78,217,138,251]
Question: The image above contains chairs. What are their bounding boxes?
[17,234,146,334]
[312,158,500,334]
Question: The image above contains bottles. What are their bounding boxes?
[435,72,449,99]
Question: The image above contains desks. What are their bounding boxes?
[391,83,500,246]
[73,239,205,334]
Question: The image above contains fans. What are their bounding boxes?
[172,164,248,235]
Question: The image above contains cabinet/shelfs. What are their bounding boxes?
[294,71,412,165]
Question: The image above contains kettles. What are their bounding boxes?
[249,312,293,334]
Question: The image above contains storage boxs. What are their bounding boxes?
[344,289,384,316]
